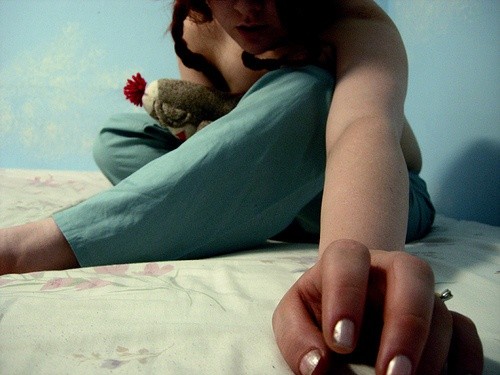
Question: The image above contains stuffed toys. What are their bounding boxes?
[123,72,242,144]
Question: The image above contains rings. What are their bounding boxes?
[434,289,453,304]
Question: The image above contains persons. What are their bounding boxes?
[1,0,486,375]
